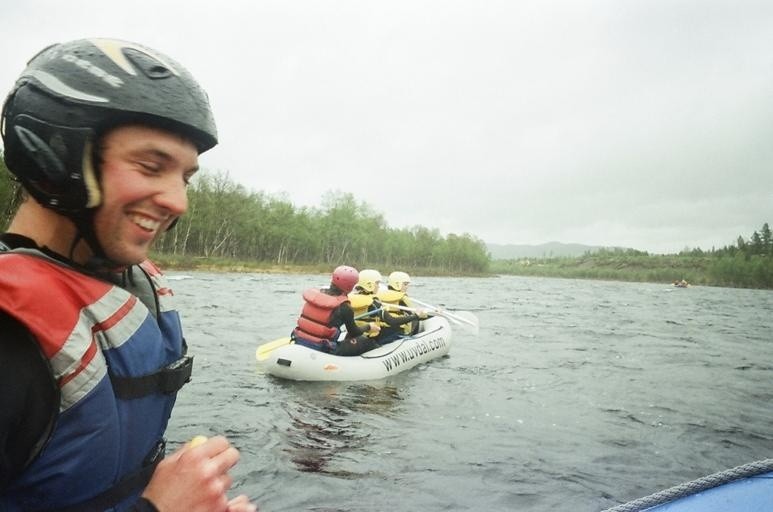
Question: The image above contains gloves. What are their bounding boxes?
[415,311,432,321]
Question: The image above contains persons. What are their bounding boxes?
[294,265,440,357]
[674,279,687,286]
[0,38,256,512]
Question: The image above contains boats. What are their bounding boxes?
[253,307,454,384]
[669,282,692,289]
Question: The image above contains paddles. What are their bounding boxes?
[407,297,479,330]
[256,306,387,359]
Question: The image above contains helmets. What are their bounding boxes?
[0,36,219,218]
[387,271,411,292]
[331,265,383,293]
